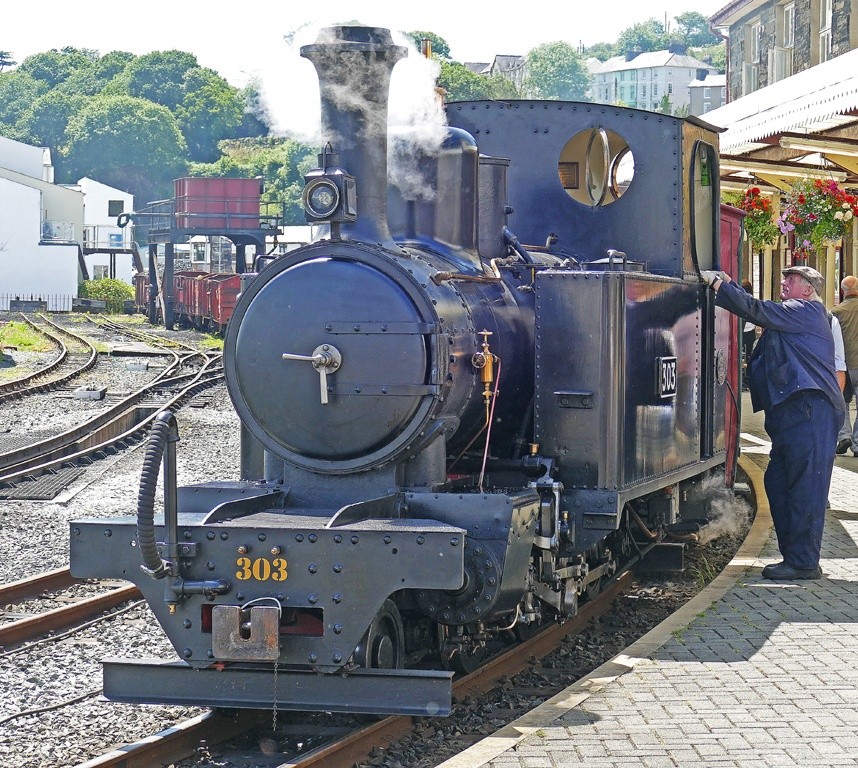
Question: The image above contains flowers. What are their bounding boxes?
[719,164,858,260]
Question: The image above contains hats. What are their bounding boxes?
[782,266,825,297]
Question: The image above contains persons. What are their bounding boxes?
[825,275,858,507]
[738,279,756,391]
[701,266,846,579]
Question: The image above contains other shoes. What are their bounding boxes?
[761,561,822,580]
[826,501,831,509]
[836,438,852,454]
[854,452,858,457]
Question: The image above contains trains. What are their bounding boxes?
[69,25,751,719]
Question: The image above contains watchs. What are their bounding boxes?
[710,275,722,288]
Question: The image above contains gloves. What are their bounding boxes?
[712,270,725,282]
[700,270,714,285]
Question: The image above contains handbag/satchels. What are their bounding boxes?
[843,365,854,403]
